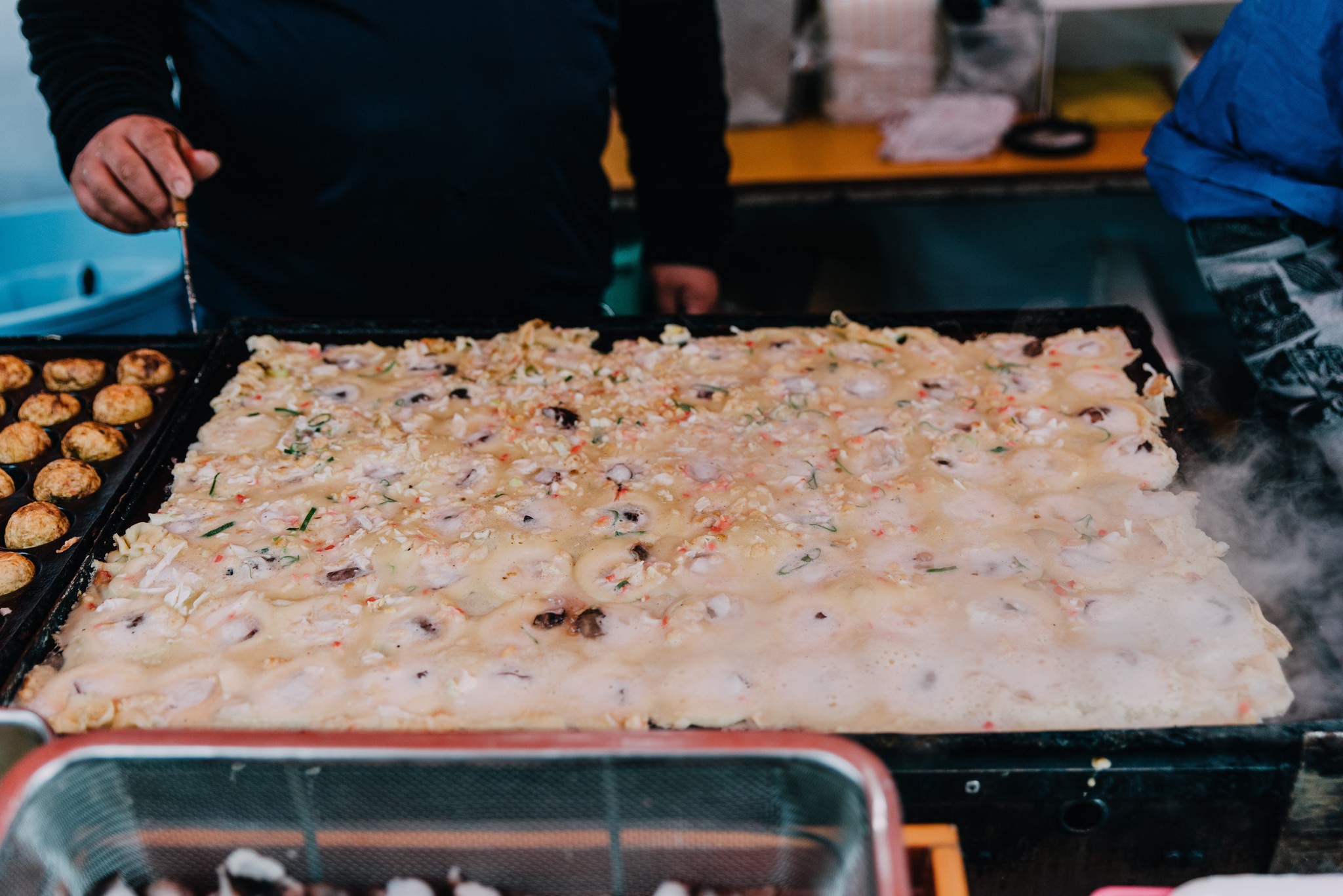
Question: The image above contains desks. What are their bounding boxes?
[602,66,1175,208]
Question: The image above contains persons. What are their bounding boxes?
[1138,0,1343,227]
[16,0,731,333]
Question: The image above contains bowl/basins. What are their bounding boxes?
[0,734,975,896]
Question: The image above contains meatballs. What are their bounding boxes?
[1,347,171,594]
